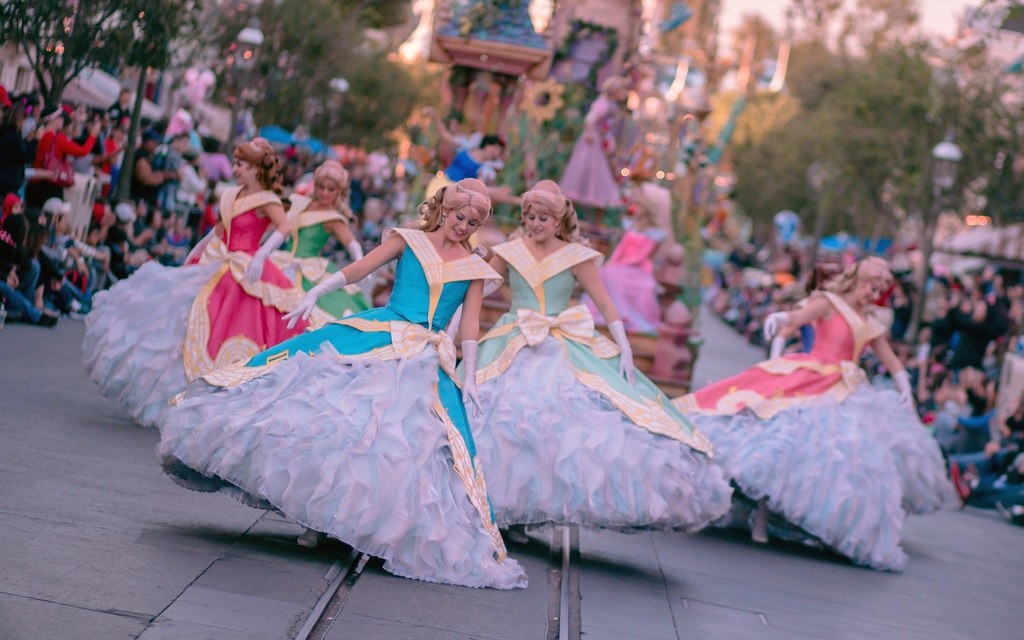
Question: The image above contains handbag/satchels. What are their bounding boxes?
[44,134,74,186]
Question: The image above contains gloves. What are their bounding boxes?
[445,303,463,343]
[349,242,372,279]
[461,340,484,417]
[245,231,284,284]
[764,312,788,340]
[281,271,346,329]
[893,371,912,411]
[607,320,635,386]
[183,226,216,265]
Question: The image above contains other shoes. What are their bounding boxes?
[21,314,34,323]
[297,528,328,548]
[38,313,58,327]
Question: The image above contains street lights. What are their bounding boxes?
[902,140,961,348]
[303,76,353,146]
[216,25,265,155]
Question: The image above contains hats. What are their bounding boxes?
[115,202,137,222]
[41,198,71,214]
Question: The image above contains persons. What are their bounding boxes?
[0,82,420,328]
[712,239,1024,531]
[591,207,659,335]
[748,255,915,545]
[447,179,637,545]
[425,112,509,211]
[560,75,627,207]
[280,177,505,549]
[172,136,292,411]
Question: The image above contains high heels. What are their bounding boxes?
[508,524,528,544]
[746,510,768,543]
